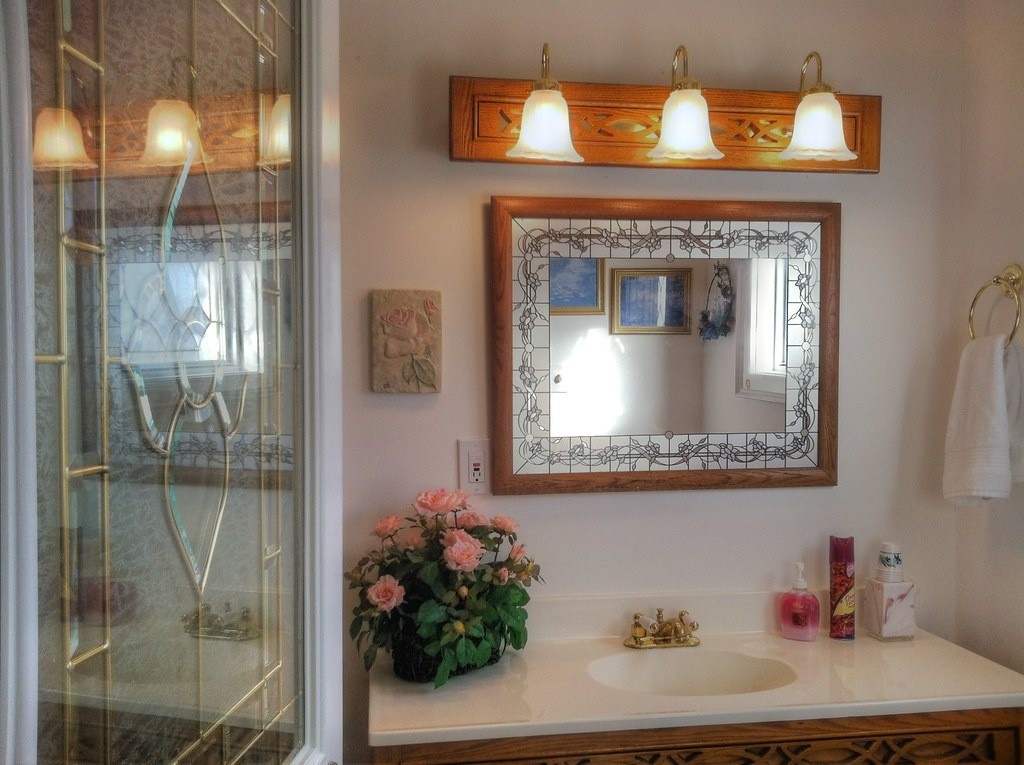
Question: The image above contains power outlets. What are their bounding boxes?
[456,438,488,496]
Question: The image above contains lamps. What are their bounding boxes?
[133,55,214,168]
[643,44,725,160]
[29,75,98,170]
[254,82,292,166]
[508,40,584,165]
[775,52,859,162]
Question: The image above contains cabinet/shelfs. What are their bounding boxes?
[369,705,1024,765]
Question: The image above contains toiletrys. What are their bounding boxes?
[828,534,855,641]
[777,561,821,642]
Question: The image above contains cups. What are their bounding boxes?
[876,541,904,585]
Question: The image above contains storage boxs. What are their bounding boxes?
[862,577,917,643]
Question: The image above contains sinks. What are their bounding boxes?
[585,645,799,697]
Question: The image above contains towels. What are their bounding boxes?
[940,332,1024,506]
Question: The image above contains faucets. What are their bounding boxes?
[653,607,689,644]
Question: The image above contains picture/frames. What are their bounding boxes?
[613,268,691,334]
[549,257,604,317]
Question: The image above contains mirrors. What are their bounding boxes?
[490,195,840,494]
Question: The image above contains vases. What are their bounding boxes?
[394,621,500,679]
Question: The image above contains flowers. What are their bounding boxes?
[699,264,735,343]
[348,484,537,668]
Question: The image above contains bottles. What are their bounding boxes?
[829,535,856,641]
